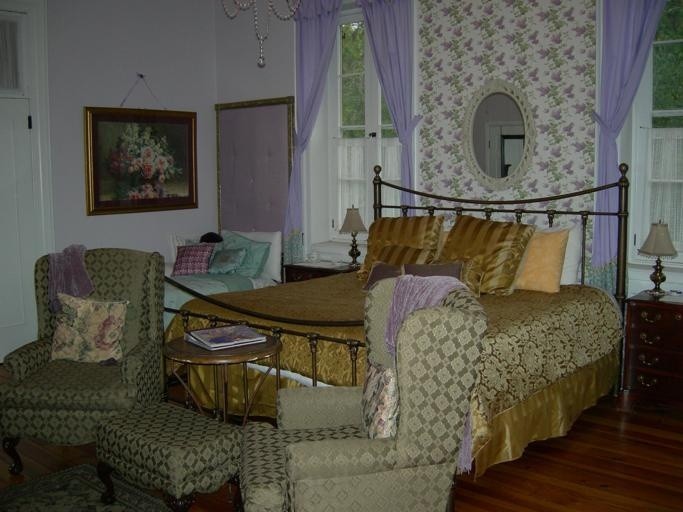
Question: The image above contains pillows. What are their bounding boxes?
[361,215,569,300]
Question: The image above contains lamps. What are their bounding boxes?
[637,220,678,296]
[336,204,366,267]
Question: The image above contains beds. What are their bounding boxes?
[164,164,630,477]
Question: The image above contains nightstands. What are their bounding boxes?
[622,291,683,414]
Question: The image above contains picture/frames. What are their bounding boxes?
[81,103,199,217]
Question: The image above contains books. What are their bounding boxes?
[183,324,266,351]
[189,324,266,347]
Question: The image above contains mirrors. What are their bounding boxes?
[462,78,535,191]
